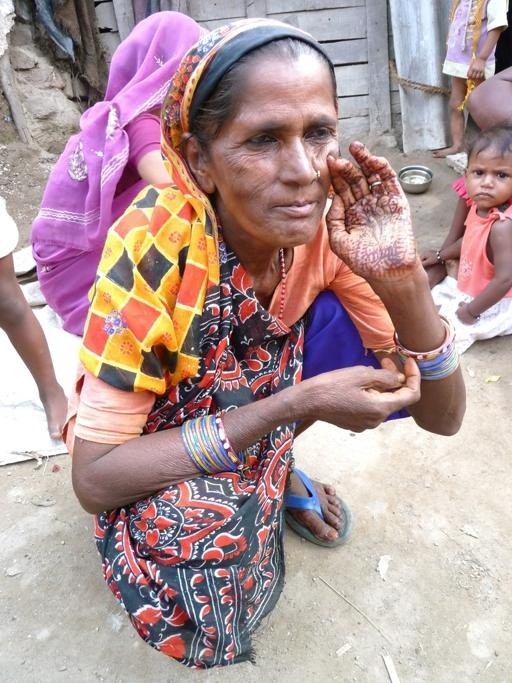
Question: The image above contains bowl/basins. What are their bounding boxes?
[396,165,432,194]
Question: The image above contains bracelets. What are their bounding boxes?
[180,407,245,475]
[388,314,462,383]
[469,311,480,320]
[435,250,446,265]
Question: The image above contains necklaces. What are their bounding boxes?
[277,247,286,320]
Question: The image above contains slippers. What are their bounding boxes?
[283,466,353,548]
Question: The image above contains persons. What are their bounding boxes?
[429,0,511,158]
[67,11,468,663]
[423,173,477,289]
[26,8,214,333]
[418,124,512,354]
[459,65,510,133]
[0,192,72,443]
